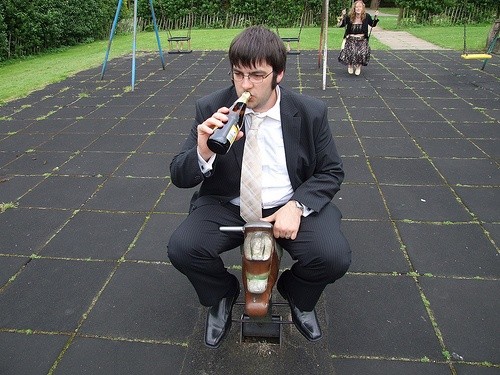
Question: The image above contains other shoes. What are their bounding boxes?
[355,67,361,75]
[347,66,353,73]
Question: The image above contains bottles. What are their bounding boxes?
[207,91,251,154]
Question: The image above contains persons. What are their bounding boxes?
[336,1,379,76]
[167,24,352,349]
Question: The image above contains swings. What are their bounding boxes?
[271,0,307,54]
[341,0,381,42]
[461,0,500,59]
[158,0,193,54]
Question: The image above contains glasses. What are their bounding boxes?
[227,65,273,82]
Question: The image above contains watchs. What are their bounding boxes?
[295,200,304,215]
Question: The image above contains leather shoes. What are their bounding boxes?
[205,273,241,348]
[277,269,323,341]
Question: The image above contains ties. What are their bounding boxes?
[240,113,264,222]
